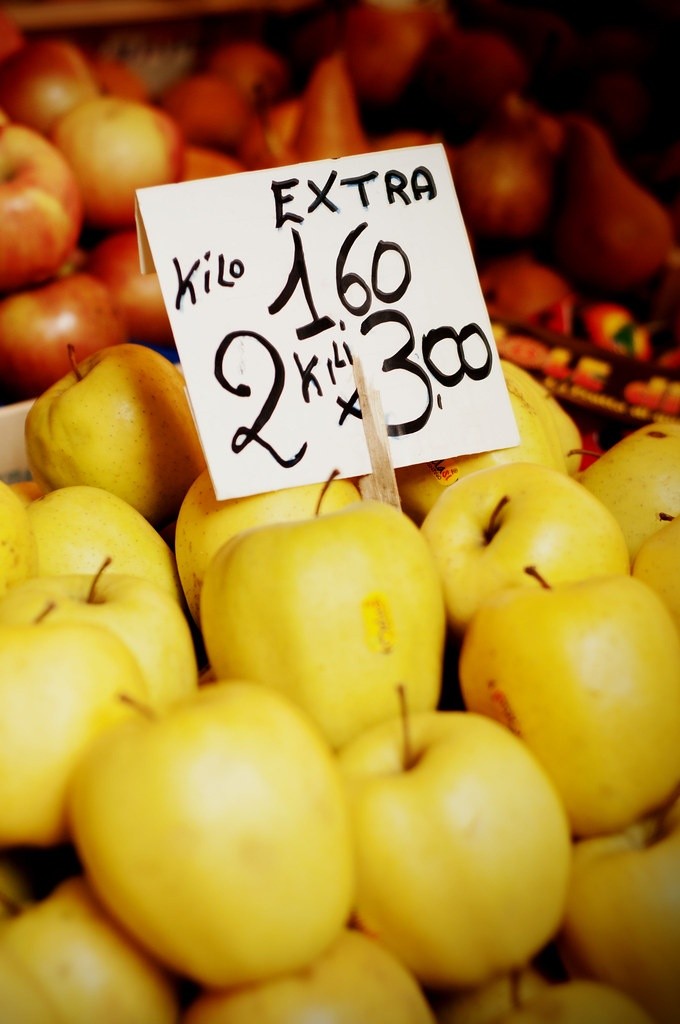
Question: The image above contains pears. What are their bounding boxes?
[231,0,669,343]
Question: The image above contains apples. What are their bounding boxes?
[0,6,313,393]
[0,351,680,1024]
